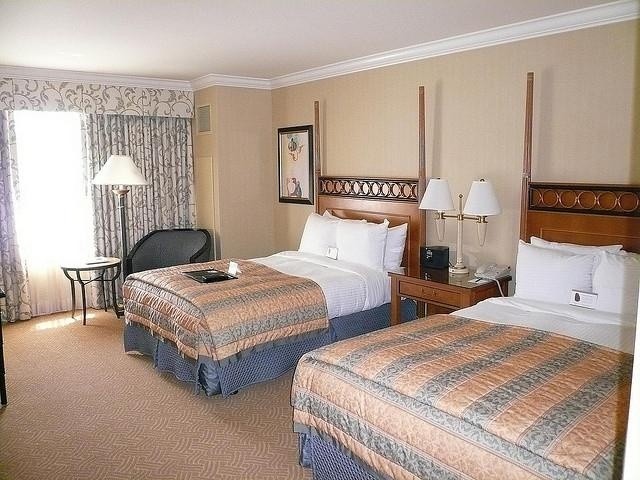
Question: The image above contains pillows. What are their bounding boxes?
[298,212,339,259]
[513,239,600,309]
[590,251,640,316]
[336,219,390,275]
[322,210,367,224]
[531,236,623,255]
[384,222,408,275]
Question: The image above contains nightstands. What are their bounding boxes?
[388,267,512,326]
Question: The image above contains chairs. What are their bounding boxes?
[124,228,212,282]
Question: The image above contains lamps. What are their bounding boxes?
[91,154,149,281]
[419,176,501,274]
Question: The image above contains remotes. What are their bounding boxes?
[201,273,228,282]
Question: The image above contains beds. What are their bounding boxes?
[291,183,640,479]
[123,175,426,397]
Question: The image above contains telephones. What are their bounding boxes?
[474,262,511,280]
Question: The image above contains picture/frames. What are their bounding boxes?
[277,124,314,205]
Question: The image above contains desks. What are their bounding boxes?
[60,256,121,325]
